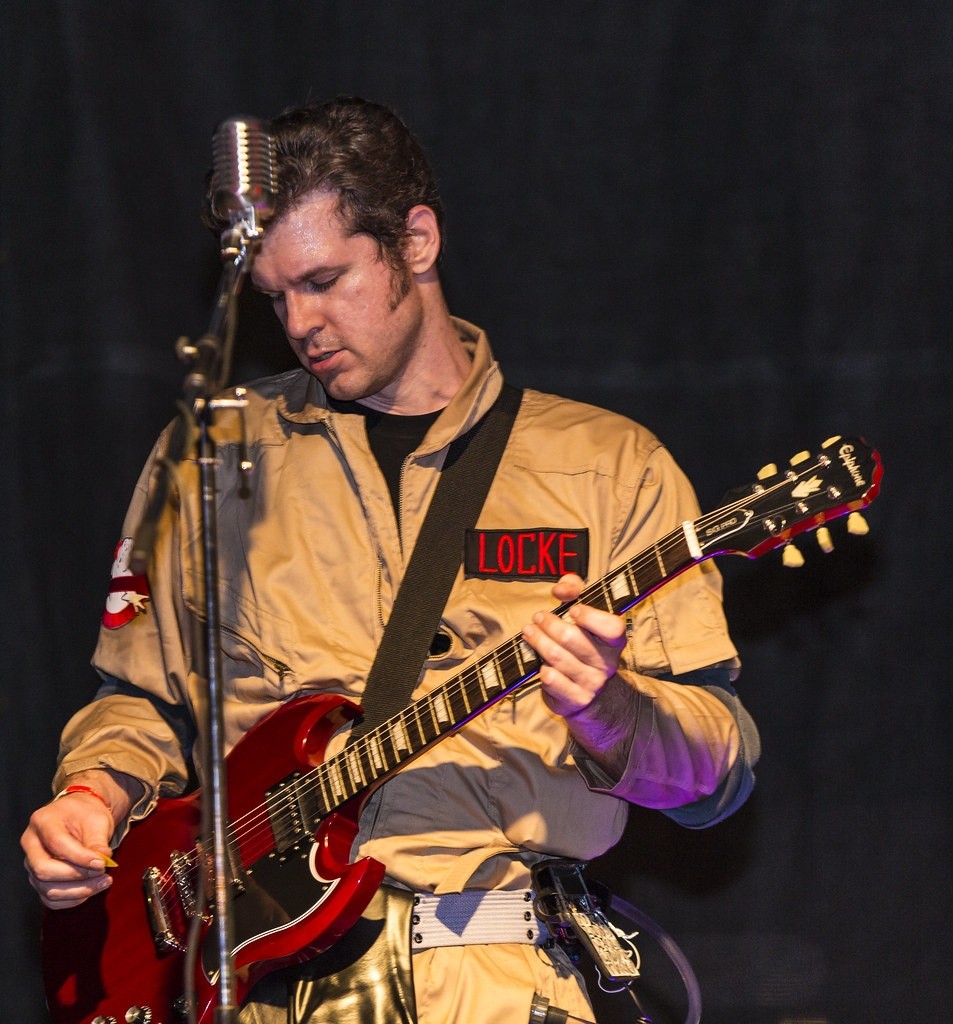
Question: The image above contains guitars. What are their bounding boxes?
[29,426,892,1024]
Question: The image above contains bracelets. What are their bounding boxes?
[52,786,112,812]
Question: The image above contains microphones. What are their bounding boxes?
[205,114,279,222]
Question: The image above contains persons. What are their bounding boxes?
[20,96,760,1023]
